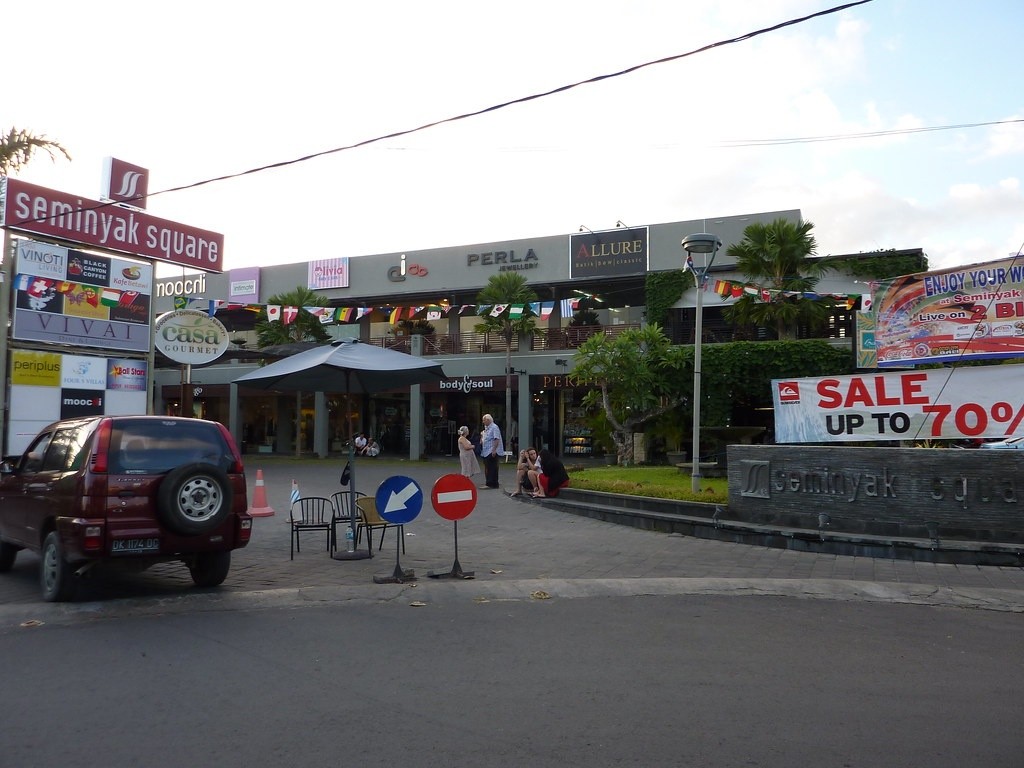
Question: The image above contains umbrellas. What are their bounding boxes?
[231,336,449,550]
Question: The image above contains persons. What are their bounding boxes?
[303,414,314,455]
[509,416,518,458]
[361,437,380,457]
[479,413,503,490]
[352,432,367,455]
[511,447,570,498]
[458,425,481,478]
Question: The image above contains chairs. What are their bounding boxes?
[289,491,405,560]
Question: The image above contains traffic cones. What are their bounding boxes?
[247,467,276,518]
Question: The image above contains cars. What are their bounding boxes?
[980,436,1024,449]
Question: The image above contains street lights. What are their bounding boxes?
[680,232,723,493]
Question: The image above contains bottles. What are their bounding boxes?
[345,525,355,553]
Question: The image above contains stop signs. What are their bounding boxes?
[430,474,477,520]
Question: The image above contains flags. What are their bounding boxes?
[704,275,872,313]
[13,273,141,308]
[382,292,597,326]
[172,297,375,323]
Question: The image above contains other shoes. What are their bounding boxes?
[511,491,522,497]
[527,492,534,497]
[478,485,490,489]
[533,494,546,498]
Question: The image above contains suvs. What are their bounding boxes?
[0,404,252,601]
